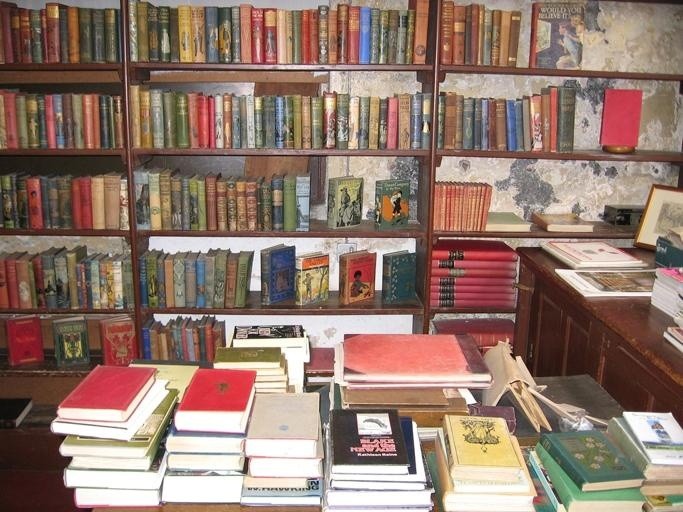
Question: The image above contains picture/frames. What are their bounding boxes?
[633,183,683,251]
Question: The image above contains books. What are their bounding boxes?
[261,243,329,306]
[543,240,683,351]
[128,0,436,65]
[435,181,595,233]
[0,1,122,63]
[0,91,124,150]
[339,249,377,303]
[0,312,682,511]
[437,87,576,149]
[430,239,518,310]
[1,245,135,309]
[139,247,255,308]
[383,250,415,303]
[0,168,410,231]
[130,86,432,149]
[439,3,584,70]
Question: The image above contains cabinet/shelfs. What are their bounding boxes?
[0,0,683,377]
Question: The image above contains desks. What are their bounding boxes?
[0,371,628,512]
[512,245,682,425]
